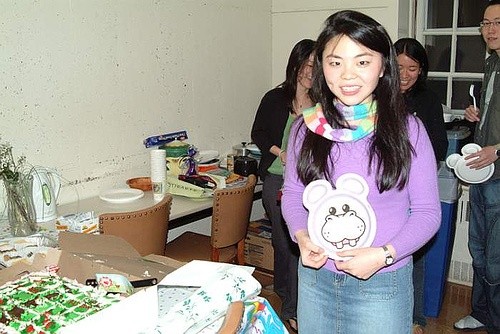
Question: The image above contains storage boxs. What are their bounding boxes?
[446,127,470,158]
[242,218,275,277]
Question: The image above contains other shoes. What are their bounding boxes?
[454,315,485,331]
[285,317,298,334]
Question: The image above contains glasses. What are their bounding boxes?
[479,19,500,31]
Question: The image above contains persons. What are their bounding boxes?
[391,36,449,334]
[455,0,500,334]
[280,10,444,334]
[251,39,319,334]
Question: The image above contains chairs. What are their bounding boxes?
[98,195,172,257]
[166,174,257,266]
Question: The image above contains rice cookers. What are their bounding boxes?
[232,141,251,157]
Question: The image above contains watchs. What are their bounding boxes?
[495,148,500,157]
[380,244,394,265]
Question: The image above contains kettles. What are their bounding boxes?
[22,166,60,223]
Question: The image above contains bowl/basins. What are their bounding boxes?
[126,177,152,191]
[247,145,261,154]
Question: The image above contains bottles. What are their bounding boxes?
[158,139,194,179]
[227,154,237,170]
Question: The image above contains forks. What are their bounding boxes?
[470,84,476,110]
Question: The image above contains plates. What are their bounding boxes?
[199,149,220,164]
[99,189,145,204]
[445,142,495,184]
[302,174,377,262]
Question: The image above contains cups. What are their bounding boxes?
[150,149,166,202]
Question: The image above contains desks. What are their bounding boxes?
[0,151,267,334]
